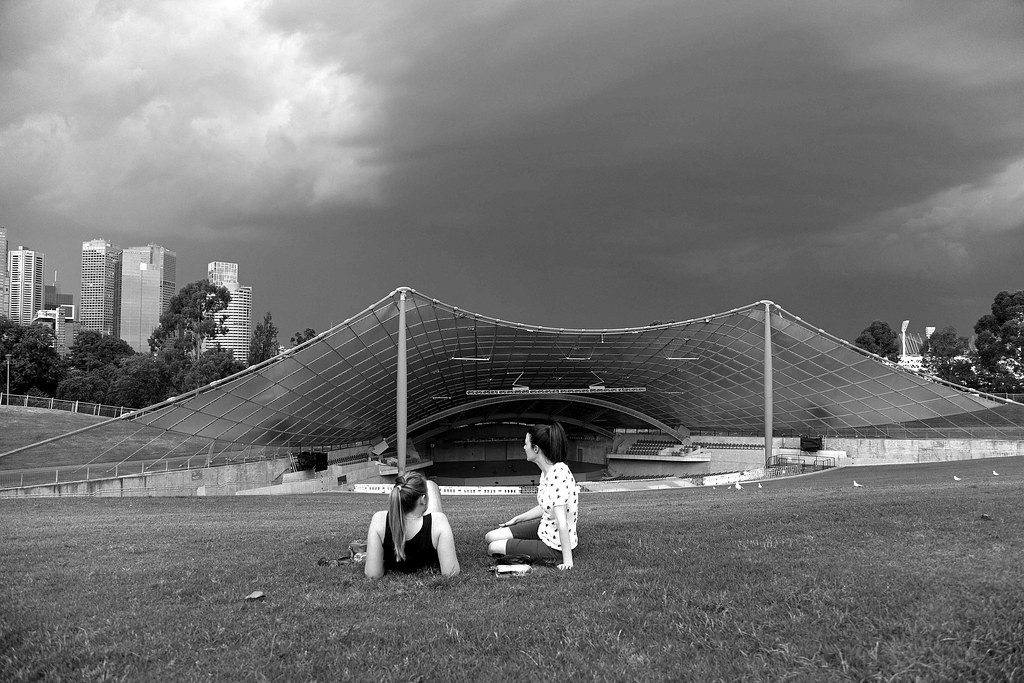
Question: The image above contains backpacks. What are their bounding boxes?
[349,538,368,561]
[497,553,536,567]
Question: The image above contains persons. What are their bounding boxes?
[363,469,461,578]
[485,421,578,571]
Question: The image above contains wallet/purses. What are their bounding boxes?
[494,564,532,577]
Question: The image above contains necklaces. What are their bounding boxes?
[405,518,419,520]
